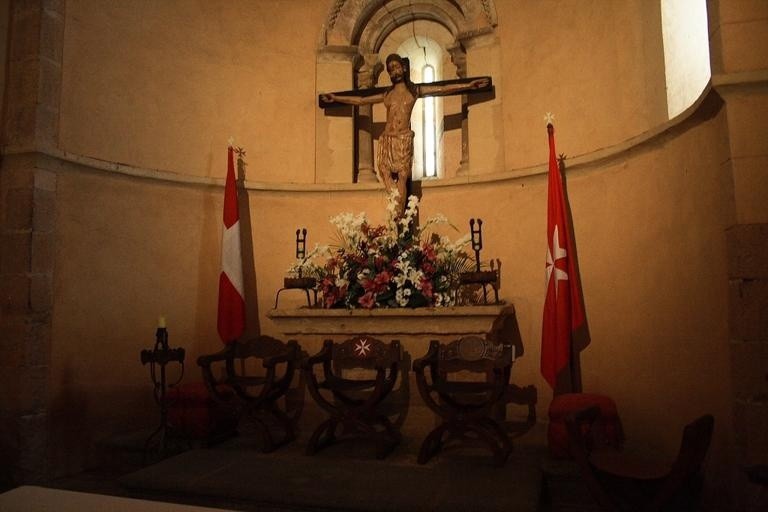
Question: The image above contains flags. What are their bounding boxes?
[538,125,591,396]
[216,146,246,344]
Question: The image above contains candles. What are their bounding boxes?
[157,315,166,327]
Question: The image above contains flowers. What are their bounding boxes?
[287,184,493,309]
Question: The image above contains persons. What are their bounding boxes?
[320,53,491,226]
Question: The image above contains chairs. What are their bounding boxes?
[412,335,536,464]
[303,335,411,460]
[593,412,715,511]
[195,333,302,455]
